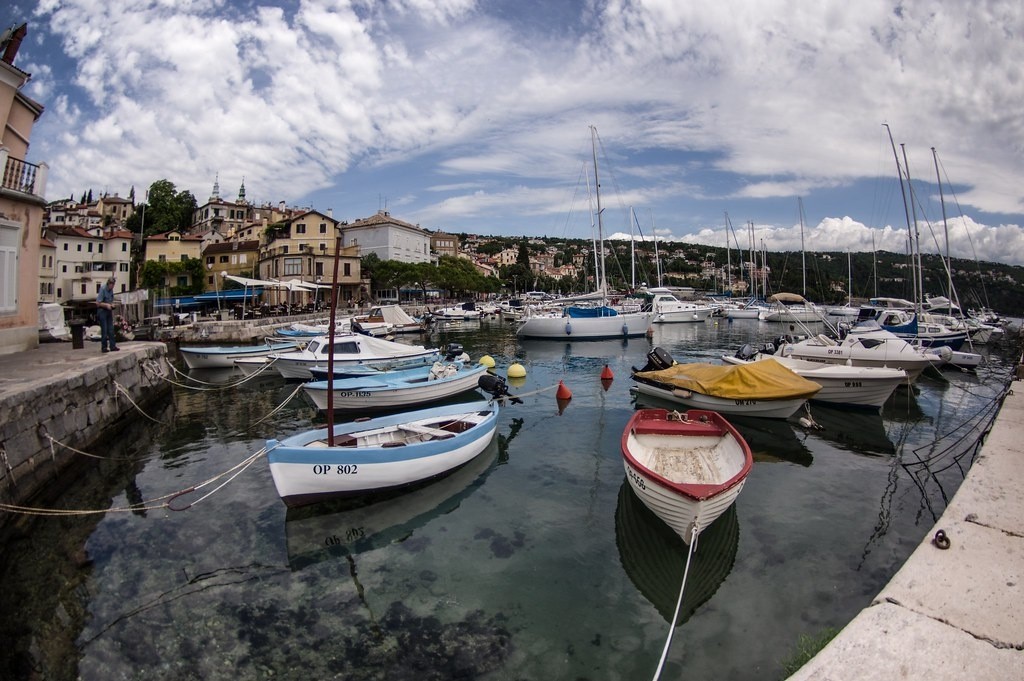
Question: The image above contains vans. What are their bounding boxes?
[526,291,545,300]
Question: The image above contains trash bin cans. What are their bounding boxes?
[69,323,86,349]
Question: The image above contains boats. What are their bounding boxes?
[267,318,470,382]
[425,299,485,319]
[265,370,523,509]
[284,418,525,621]
[630,345,824,419]
[482,297,522,320]
[301,357,489,418]
[722,345,905,412]
[621,409,752,545]
[179,298,427,377]
[761,299,941,388]
[616,474,740,629]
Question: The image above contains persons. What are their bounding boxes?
[233,298,325,319]
[348,298,364,308]
[95,276,120,353]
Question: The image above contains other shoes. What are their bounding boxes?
[102,350,110,353]
[110,347,120,351]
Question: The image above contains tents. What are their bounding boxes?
[269,277,312,311]
[289,278,341,313]
[223,275,292,320]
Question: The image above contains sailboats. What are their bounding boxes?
[629,206,714,324]
[707,211,768,318]
[515,123,659,341]
[761,194,862,324]
[837,121,1014,351]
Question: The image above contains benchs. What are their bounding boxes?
[635,419,722,452]
[390,381,408,385]
[397,423,459,440]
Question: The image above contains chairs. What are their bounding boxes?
[233,303,314,319]
